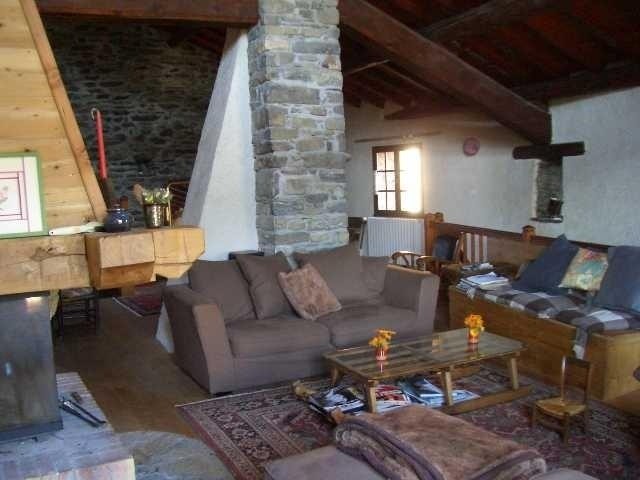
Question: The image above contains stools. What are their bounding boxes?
[54,286,101,343]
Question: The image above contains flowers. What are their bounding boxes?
[463,313,485,338]
[367,328,396,351]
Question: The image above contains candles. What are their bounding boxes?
[91,108,108,181]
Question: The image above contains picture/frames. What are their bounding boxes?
[0,151,49,240]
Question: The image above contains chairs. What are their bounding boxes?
[391,228,469,306]
[529,354,597,449]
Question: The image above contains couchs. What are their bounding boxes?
[162,257,441,398]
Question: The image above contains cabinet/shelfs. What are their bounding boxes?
[133,224,206,280]
[85,225,157,291]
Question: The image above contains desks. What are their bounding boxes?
[440,260,516,287]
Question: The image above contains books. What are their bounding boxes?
[308,374,481,420]
[460,272,511,286]
[460,278,479,286]
[455,282,479,293]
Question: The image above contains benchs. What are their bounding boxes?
[447,224,640,403]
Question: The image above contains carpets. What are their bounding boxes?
[169,355,639,479]
[111,279,164,319]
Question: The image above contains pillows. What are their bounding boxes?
[558,246,610,292]
[277,262,343,321]
[187,259,256,326]
[515,234,574,293]
[591,244,639,316]
[363,256,390,293]
[236,251,292,320]
[292,240,383,305]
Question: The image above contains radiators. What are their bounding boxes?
[366,217,427,269]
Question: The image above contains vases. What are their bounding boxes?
[375,344,389,361]
[467,327,479,344]
